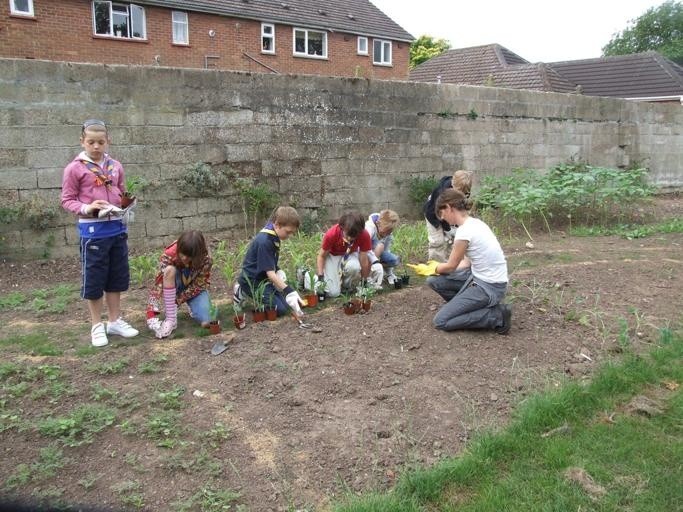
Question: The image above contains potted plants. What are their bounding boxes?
[393,253,410,289]
[231,300,254,329]
[340,284,376,314]
[207,300,220,334]
[243,269,283,323]
[304,267,333,308]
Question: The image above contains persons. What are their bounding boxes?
[406,189,513,335]
[232,206,305,318]
[315,213,372,299]
[422,169,473,282]
[145,229,214,339]
[59,120,141,347]
[356,209,401,289]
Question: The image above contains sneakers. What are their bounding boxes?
[106,318,140,339]
[154,318,178,339]
[231,280,248,313]
[295,264,310,294]
[89,321,109,348]
[494,303,513,335]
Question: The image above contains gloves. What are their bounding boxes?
[365,261,383,287]
[443,225,457,247]
[312,274,329,296]
[282,286,306,319]
[96,197,136,226]
[275,269,288,285]
[404,259,442,277]
[146,315,163,333]
[387,272,399,285]
